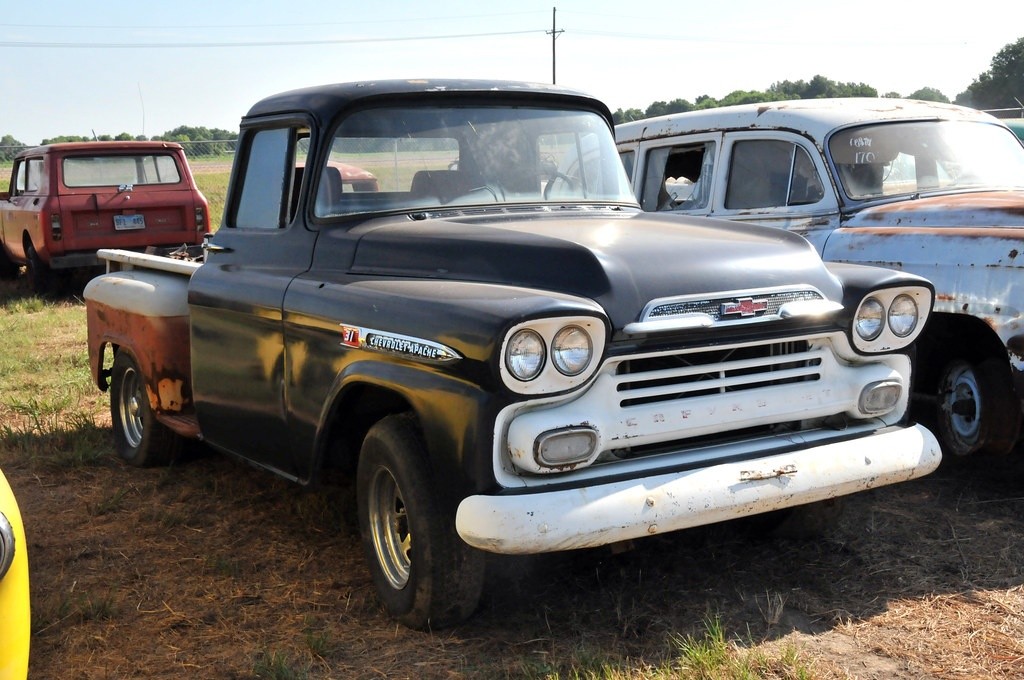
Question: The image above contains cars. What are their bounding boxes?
[885,117,1024,184]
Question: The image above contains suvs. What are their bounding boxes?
[542,95,1024,470]
[0,140,215,299]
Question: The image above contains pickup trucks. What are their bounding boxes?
[84,76,944,629]
